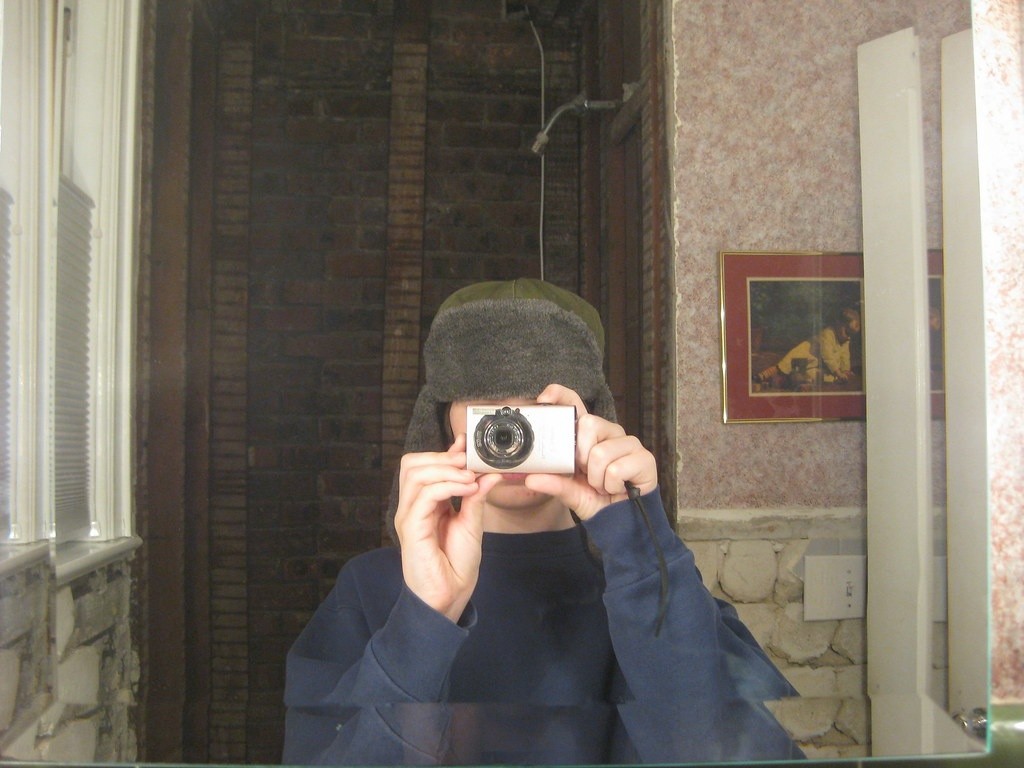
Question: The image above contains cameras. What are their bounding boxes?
[465,404,578,473]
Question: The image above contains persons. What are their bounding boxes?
[753,309,860,393]
[282,276,809,761]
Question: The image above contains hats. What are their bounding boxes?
[386,279,618,555]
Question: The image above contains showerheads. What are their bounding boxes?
[531,132,548,157]
